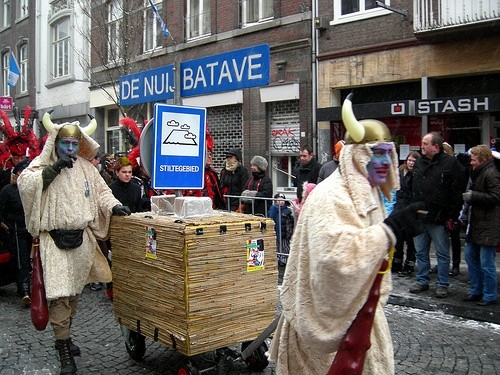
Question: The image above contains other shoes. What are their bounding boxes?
[54,337,80,355]
[449,269,460,276]
[429,266,439,274]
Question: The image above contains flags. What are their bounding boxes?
[149,0,170,39]
[7,50,21,89]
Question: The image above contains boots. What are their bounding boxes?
[21,282,32,306]
[53,340,76,375]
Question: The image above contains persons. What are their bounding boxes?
[268,192,295,267]
[217,148,251,213]
[1,158,33,306]
[265,118,400,375]
[294,143,323,203]
[432,138,468,276]
[149,121,225,210]
[409,129,454,298]
[315,139,347,183]
[239,154,274,216]
[389,147,423,277]
[16,120,132,375]
[457,143,500,306]
[90,148,150,300]
[0,146,28,188]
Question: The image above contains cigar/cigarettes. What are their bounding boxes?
[70,157,75,163]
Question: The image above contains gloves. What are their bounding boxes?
[61,157,74,169]
[462,190,472,201]
[384,196,429,241]
[112,205,131,216]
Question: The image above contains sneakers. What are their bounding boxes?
[435,283,448,297]
[408,280,429,292]
[480,298,498,305]
[398,266,412,276]
[464,291,481,300]
[90,284,101,291]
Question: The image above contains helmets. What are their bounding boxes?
[55,125,82,138]
[344,118,392,143]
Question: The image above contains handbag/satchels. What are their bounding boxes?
[50,228,84,249]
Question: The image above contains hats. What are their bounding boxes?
[223,148,242,160]
[250,155,268,171]
[334,139,345,159]
[274,193,291,206]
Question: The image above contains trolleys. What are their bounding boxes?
[124,311,284,375]
